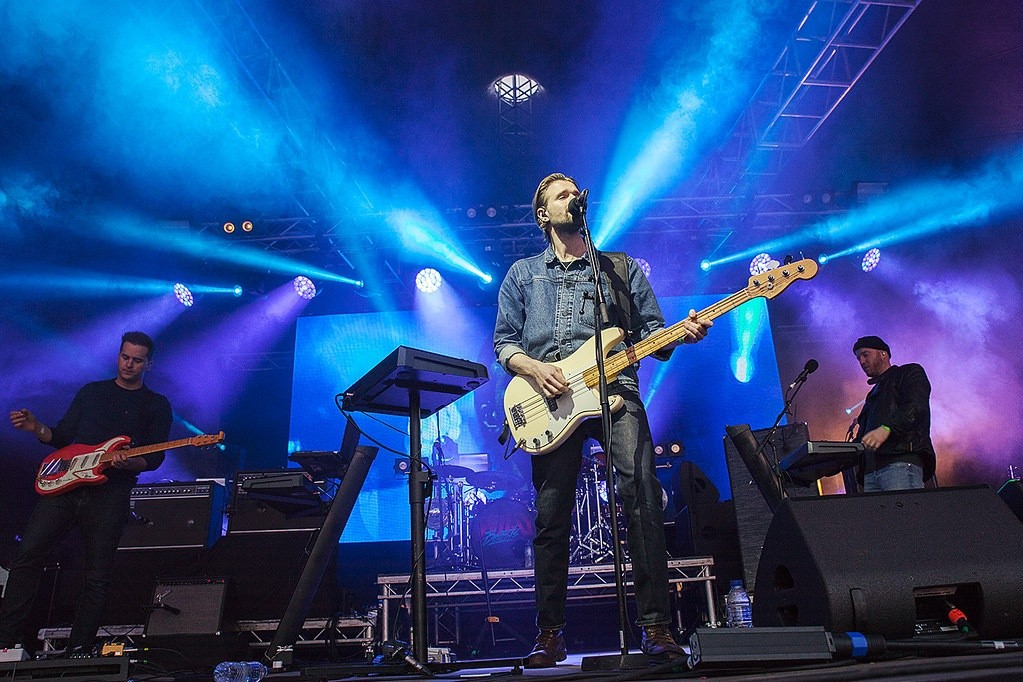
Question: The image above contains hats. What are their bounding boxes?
[853,335,891,359]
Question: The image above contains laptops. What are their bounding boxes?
[292,413,361,467]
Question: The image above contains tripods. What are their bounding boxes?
[424,453,480,573]
[568,455,629,566]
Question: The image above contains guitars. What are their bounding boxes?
[34,431,225,498]
[503,246,820,456]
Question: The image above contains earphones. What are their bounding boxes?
[882,352,885,356]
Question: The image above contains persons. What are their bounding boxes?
[0,332,174,657]
[853,335,937,492]
[494,173,713,667]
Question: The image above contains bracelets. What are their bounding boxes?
[882,425,890,433]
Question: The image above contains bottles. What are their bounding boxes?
[213,661,270,682]
[526,540,534,568]
[727,580,753,627]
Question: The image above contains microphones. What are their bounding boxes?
[569,189,589,216]
[433,442,444,456]
[161,604,181,615]
[787,359,819,392]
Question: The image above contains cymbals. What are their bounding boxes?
[466,471,525,491]
[425,465,474,479]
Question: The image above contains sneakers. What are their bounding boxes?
[523,628,567,669]
[638,624,686,661]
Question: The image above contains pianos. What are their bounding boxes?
[748,440,877,495]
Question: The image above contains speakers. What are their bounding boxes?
[114,467,330,642]
[669,423,1023,641]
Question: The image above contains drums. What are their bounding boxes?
[592,505,623,521]
[469,498,536,567]
[588,452,608,479]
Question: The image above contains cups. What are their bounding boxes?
[1008,464,1021,480]
